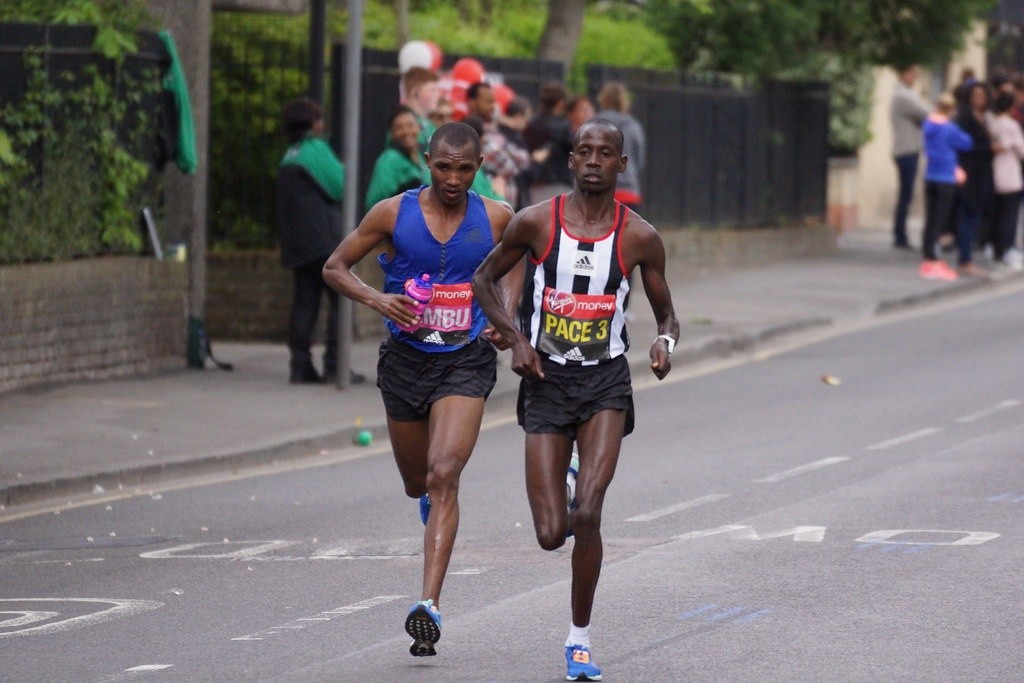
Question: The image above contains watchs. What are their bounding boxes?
[654,336,675,357]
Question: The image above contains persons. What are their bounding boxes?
[891,61,1024,281]
[362,68,647,346]
[322,123,526,656]
[472,118,681,680]
[272,100,364,385]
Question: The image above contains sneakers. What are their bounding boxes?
[565,643,602,682]
[919,259,959,282]
[419,492,432,527]
[404,598,442,656]
[564,452,580,538]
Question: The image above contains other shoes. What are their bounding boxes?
[288,359,366,386]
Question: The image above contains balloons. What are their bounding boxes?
[397,40,514,121]
